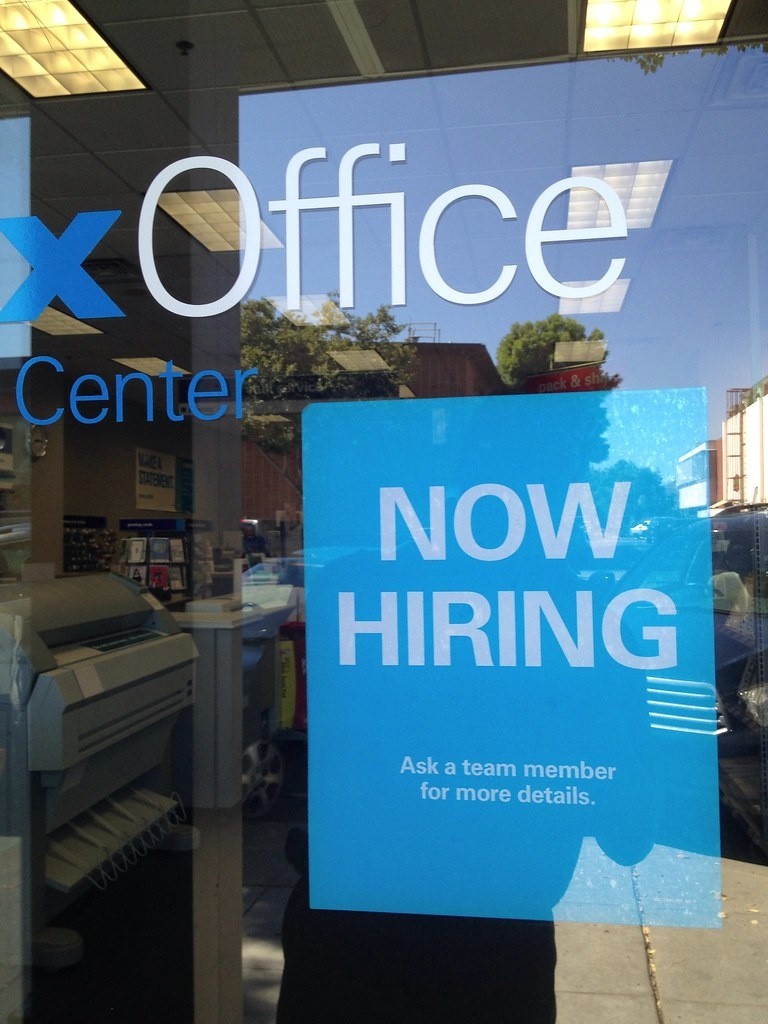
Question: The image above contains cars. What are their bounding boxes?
[238,548,371,620]
[581,539,667,606]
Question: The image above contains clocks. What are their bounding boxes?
[25,420,49,459]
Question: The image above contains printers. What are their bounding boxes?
[0,571,199,965]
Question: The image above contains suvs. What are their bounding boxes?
[591,502,767,744]
